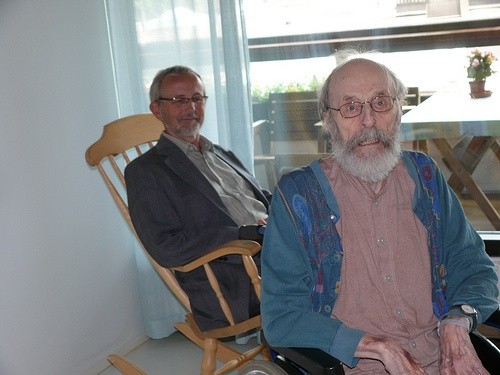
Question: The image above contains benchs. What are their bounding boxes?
[252,87,419,192]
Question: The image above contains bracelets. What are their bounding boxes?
[436,319,470,332]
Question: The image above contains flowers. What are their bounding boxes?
[466,48,498,82]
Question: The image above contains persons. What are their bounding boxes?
[260,57,500,375]
[125,66,272,332]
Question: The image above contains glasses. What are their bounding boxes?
[328,95,397,118]
[159,95,208,106]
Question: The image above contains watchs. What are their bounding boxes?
[450,304,479,333]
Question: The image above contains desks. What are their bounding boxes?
[399,83,500,230]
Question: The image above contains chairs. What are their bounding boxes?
[86,113,269,375]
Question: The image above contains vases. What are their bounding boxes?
[469,81,492,97]
